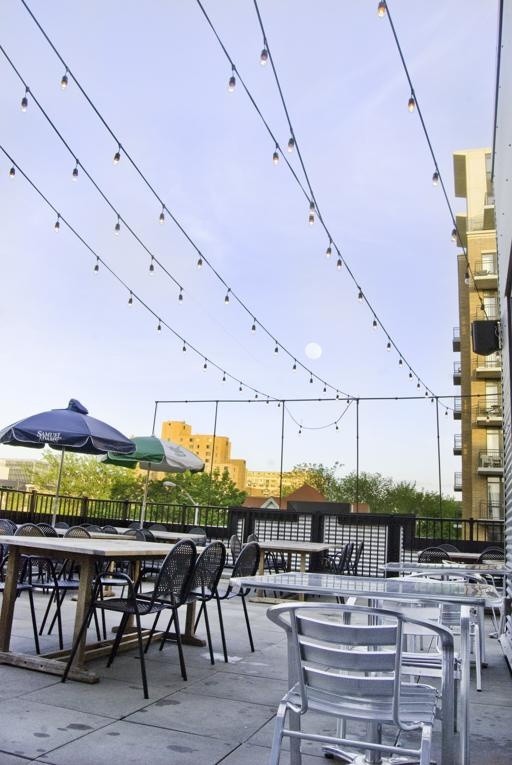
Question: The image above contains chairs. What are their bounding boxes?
[1,514,512,703]
[266,603,456,763]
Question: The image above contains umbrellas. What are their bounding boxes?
[0,398,137,528]
[102,434,205,530]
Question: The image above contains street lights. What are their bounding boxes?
[163,481,199,525]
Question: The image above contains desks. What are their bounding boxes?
[227,568,487,758]
